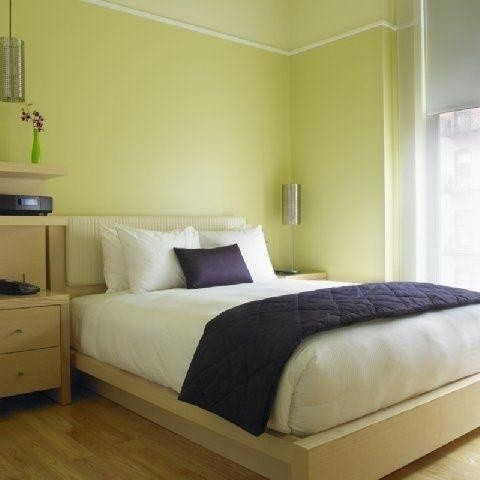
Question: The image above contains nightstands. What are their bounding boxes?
[0,290,72,406]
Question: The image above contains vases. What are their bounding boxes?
[31,128,41,163]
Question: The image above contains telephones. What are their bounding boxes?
[0,279,41,295]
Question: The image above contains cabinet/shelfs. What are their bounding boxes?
[0,162,68,226]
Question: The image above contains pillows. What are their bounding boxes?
[98,223,279,296]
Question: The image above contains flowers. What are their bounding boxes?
[18,100,46,133]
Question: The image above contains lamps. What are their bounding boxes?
[282,181,302,273]
[1,0,25,103]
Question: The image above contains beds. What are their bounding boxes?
[48,215,480,480]
